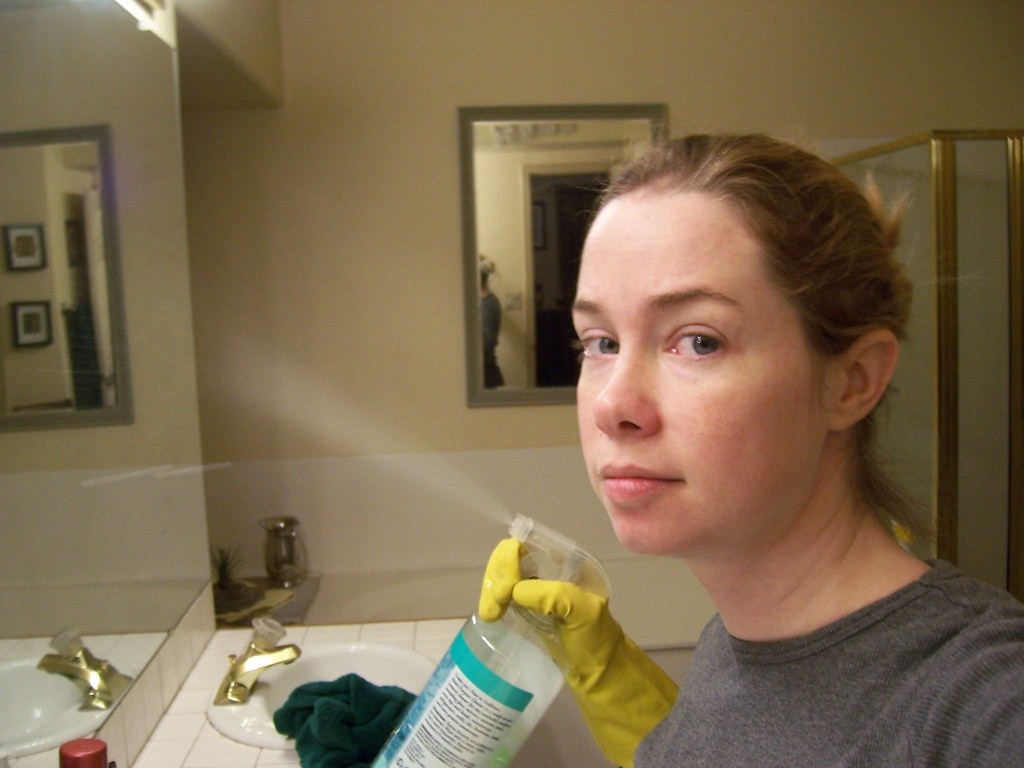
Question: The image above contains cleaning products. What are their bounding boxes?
[371,511,617,768]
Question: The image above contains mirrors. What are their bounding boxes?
[1,122,134,434]
[456,103,669,409]
[0,1,213,768]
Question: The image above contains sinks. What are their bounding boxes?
[0,656,117,760]
[203,640,439,752]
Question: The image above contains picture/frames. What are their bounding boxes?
[12,303,52,349]
[532,201,549,254]
[6,225,49,272]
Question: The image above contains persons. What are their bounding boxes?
[479,255,505,388]
[476,132,1024,768]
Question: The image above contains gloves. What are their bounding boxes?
[478,538,681,767]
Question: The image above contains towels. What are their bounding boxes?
[272,671,419,768]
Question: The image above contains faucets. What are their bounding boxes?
[212,614,303,707]
[36,624,136,714]
